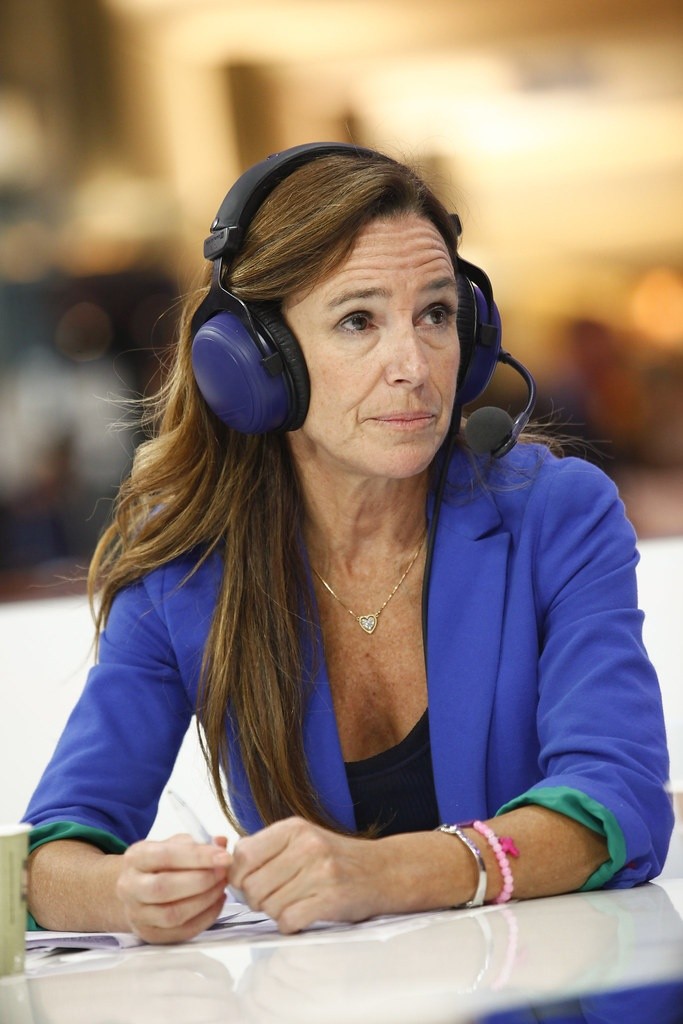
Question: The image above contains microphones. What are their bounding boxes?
[462,348,537,459]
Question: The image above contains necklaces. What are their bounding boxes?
[304,527,429,635]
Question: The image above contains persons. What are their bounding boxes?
[21,140,680,1024]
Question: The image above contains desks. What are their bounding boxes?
[0,879,683,1024]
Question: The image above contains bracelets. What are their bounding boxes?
[433,821,488,909]
[455,819,520,905]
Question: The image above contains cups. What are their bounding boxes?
[0,823,34,980]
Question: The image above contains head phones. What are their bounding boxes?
[189,142,502,440]
